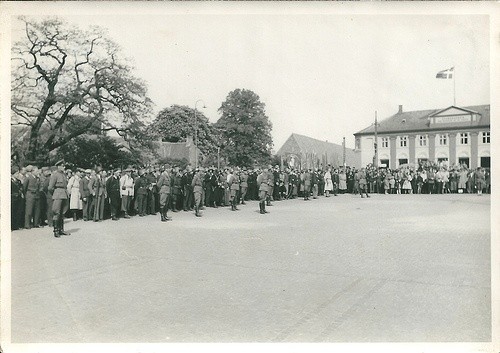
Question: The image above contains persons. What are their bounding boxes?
[11,153,19,174]
[11,159,255,237]
[269,165,359,201]
[257,165,271,214]
[357,168,369,198]
[365,164,490,196]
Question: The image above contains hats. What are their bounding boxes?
[55,159,65,166]
[149,168,156,174]
[140,169,147,177]
[164,164,171,169]
[96,166,103,174]
[268,165,273,171]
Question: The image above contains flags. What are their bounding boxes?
[436,67,454,79]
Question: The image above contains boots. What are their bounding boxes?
[195,205,202,217]
[53,219,59,236]
[59,218,68,235]
[360,191,364,198]
[307,191,310,200]
[231,201,235,211]
[159,209,164,221]
[263,203,268,213]
[259,203,264,214]
[304,190,307,200]
[365,192,369,197]
[233,201,238,210]
[164,208,170,220]
